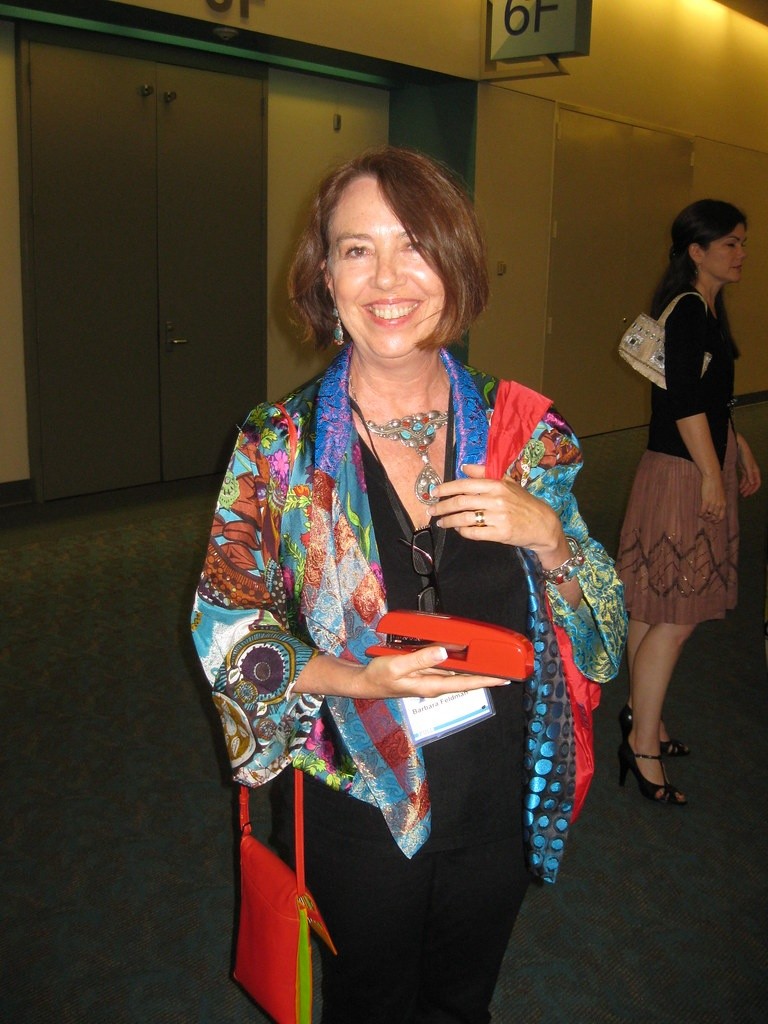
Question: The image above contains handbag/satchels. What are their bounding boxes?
[230,835,338,1024]
[618,293,712,390]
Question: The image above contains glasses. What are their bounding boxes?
[399,528,442,613]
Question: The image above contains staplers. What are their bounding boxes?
[364,611,535,681]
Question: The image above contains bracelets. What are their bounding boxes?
[543,535,585,585]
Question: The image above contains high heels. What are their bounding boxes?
[618,739,687,805]
[619,704,690,757]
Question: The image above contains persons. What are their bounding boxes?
[617,199,762,804]
[189,150,627,1024]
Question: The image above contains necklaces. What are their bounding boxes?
[351,379,448,504]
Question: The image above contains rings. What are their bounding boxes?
[707,511,712,516]
[475,513,486,527]
